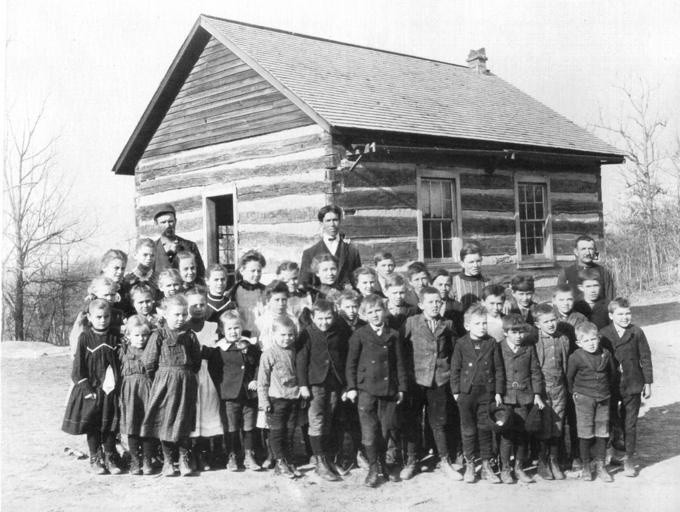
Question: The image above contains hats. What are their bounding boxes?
[153,204,176,220]
[487,404,514,435]
[525,405,545,433]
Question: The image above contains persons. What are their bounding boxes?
[309,252,346,303]
[352,266,379,298]
[451,303,507,485]
[300,203,363,291]
[209,311,263,472]
[117,314,163,475]
[481,284,512,344]
[61,298,123,474]
[574,268,628,449]
[498,313,543,484]
[185,287,220,471]
[374,251,397,290]
[400,283,464,482]
[447,243,496,309]
[406,261,432,296]
[275,260,312,323]
[121,287,161,331]
[226,251,267,339]
[509,273,539,326]
[347,295,407,486]
[422,268,463,469]
[549,283,591,471]
[69,278,126,358]
[141,296,202,478]
[296,302,353,481]
[120,237,166,313]
[172,250,208,297]
[337,290,376,475]
[253,280,298,352]
[204,264,236,323]
[529,303,572,481]
[158,268,187,308]
[380,274,425,474]
[257,316,303,479]
[598,297,654,476]
[153,204,207,279]
[558,236,615,303]
[89,249,128,295]
[566,321,621,483]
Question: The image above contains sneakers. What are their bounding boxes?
[288,464,302,477]
[227,452,238,472]
[190,448,197,472]
[440,458,463,481]
[197,450,211,471]
[315,455,339,481]
[243,450,261,471]
[129,451,140,475]
[513,459,534,483]
[90,454,105,475]
[382,463,402,483]
[582,461,592,481]
[623,458,635,477]
[563,469,580,479]
[357,451,370,469]
[572,458,582,472]
[161,452,175,476]
[499,462,515,484]
[104,452,122,475]
[611,439,625,451]
[595,461,613,482]
[262,458,277,469]
[481,460,501,483]
[274,458,294,479]
[463,456,475,483]
[400,460,421,480]
[610,449,626,465]
[179,450,192,476]
[328,461,349,476]
[143,456,152,475]
[364,460,384,488]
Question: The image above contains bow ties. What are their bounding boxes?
[328,237,337,241]
